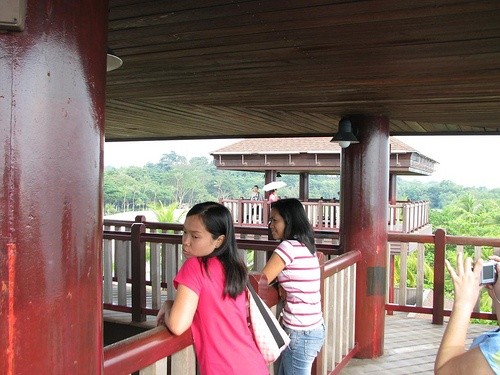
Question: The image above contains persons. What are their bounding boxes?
[152,202,269,375]
[249,185,263,223]
[269,189,278,202]
[249,197,325,375]
[433,250,500,375]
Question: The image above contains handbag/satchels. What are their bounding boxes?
[246,274,292,366]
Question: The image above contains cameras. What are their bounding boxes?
[479,263,496,284]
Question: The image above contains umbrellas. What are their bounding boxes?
[262,181,287,192]
